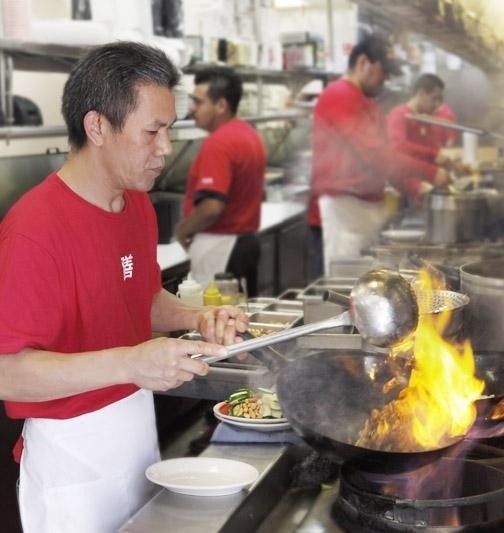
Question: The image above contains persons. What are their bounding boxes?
[309,32,434,279]
[174,64,265,299]
[386,74,458,163]
[1,38,249,530]
[427,103,457,146]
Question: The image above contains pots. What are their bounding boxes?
[233,327,477,470]
[458,254,503,352]
[424,191,489,246]
[473,363,504,419]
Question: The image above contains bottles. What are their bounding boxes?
[176,269,240,308]
[208,15,285,74]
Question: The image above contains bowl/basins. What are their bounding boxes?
[290,148,312,184]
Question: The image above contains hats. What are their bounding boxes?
[354,39,403,75]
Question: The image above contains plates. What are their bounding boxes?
[211,400,292,432]
[144,454,257,498]
[381,229,423,242]
[173,73,196,120]
[213,401,289,422]
